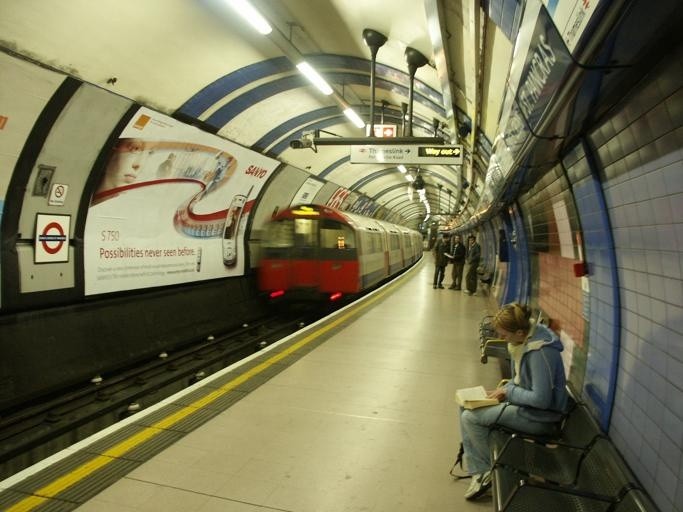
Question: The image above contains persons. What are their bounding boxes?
[448,235,467,290]
[463,236,482,296]
[458,302,570,500]
[91,138,159,207]
[156,152,176,178]
[432,233,451,290]
[215,162,223,175]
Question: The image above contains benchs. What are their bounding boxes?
[487,379,661,511]
[478,302,553,365]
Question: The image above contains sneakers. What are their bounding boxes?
[433,284,472,294]
[451,466,470,478]
[464,471,493,500]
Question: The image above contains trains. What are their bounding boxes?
[256,204,423,300]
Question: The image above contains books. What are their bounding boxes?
[454,385,500,411]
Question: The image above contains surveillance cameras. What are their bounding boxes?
[289,138,313,149]
